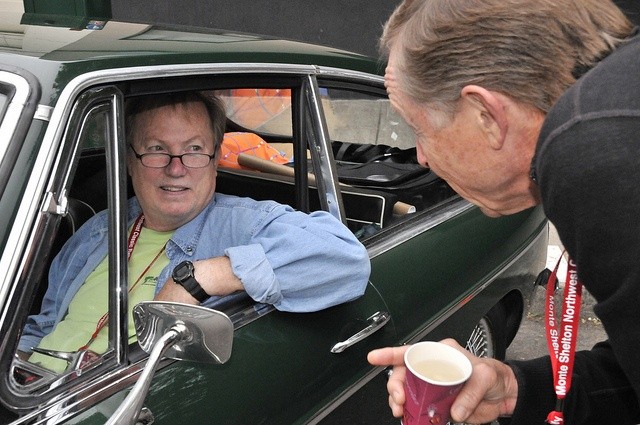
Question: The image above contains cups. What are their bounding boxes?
[401,341,471,425]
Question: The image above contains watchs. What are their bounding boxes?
[172,260,211,303]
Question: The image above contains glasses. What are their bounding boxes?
[128,140,218,168]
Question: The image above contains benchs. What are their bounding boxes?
[216,166,394,234]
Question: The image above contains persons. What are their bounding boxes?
[13,90,372,374]
[367,0,640,425]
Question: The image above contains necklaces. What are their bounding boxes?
[78,214,169,352]
[545,249,582,425]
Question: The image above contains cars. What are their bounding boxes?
[1,12,550,425]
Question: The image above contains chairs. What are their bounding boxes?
[61,198,96,235]
